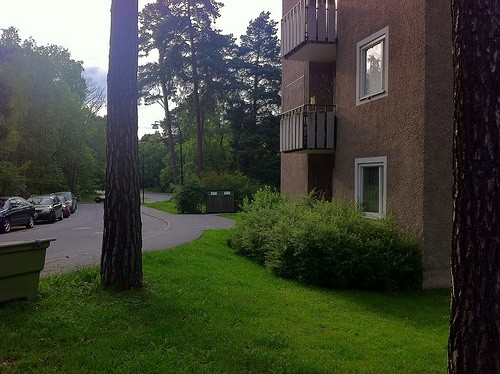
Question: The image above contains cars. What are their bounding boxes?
[27,195,64,223]
[94,195,105,203]
[50,192,77,213]
[58,195,70,218]
[0,196,38,232]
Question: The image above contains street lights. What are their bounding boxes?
[150,121,183,185]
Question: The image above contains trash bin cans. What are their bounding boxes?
[0,238,57,312]
[206,188,235,213]
